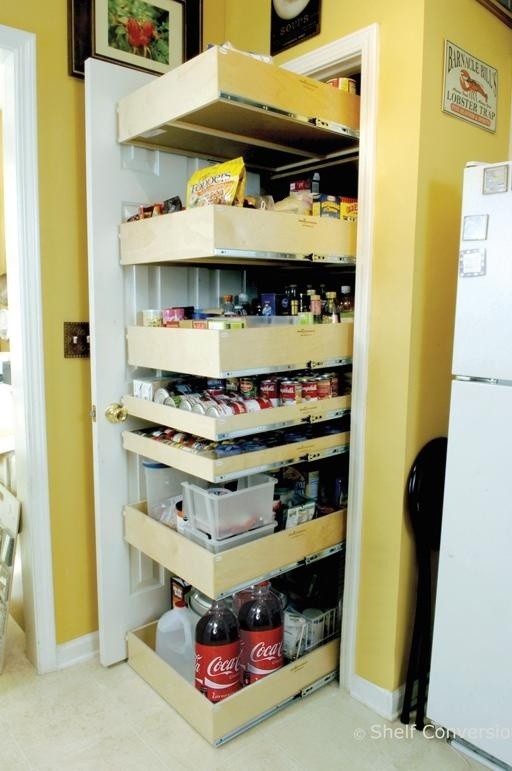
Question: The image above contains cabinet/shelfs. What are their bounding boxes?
[113,42,370,748]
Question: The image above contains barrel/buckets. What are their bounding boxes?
[154,601,201,688]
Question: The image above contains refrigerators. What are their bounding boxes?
[422,159,511,771]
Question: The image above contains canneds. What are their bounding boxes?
[173,307,195,320]
[163,309,176,324]
[142,309,162,327]
[152,372,339,418]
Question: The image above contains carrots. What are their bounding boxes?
[127,17,156,48]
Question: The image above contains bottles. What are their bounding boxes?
[219,291,273,325]
[283,282,353,326]
[193,306,207,321]
[194,578,284,704]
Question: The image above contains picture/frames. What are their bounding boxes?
[66,0,205,80]
